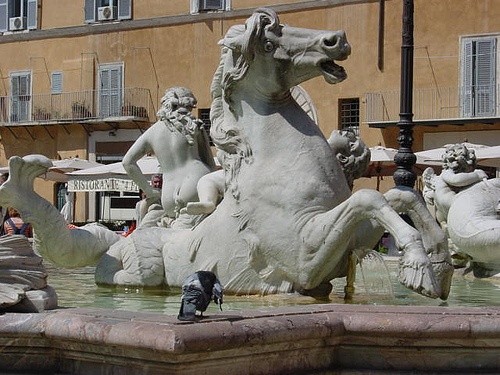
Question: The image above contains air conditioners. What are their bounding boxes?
[8,17,27,31]
[98,6,117,20]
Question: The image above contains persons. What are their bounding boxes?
[179,129,371,215]
[433,145,488,213]
[120,86,220,219]
[4,208,31,237]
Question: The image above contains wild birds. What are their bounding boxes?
[177,271,223,321]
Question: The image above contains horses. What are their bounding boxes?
[1,6,455,300]
